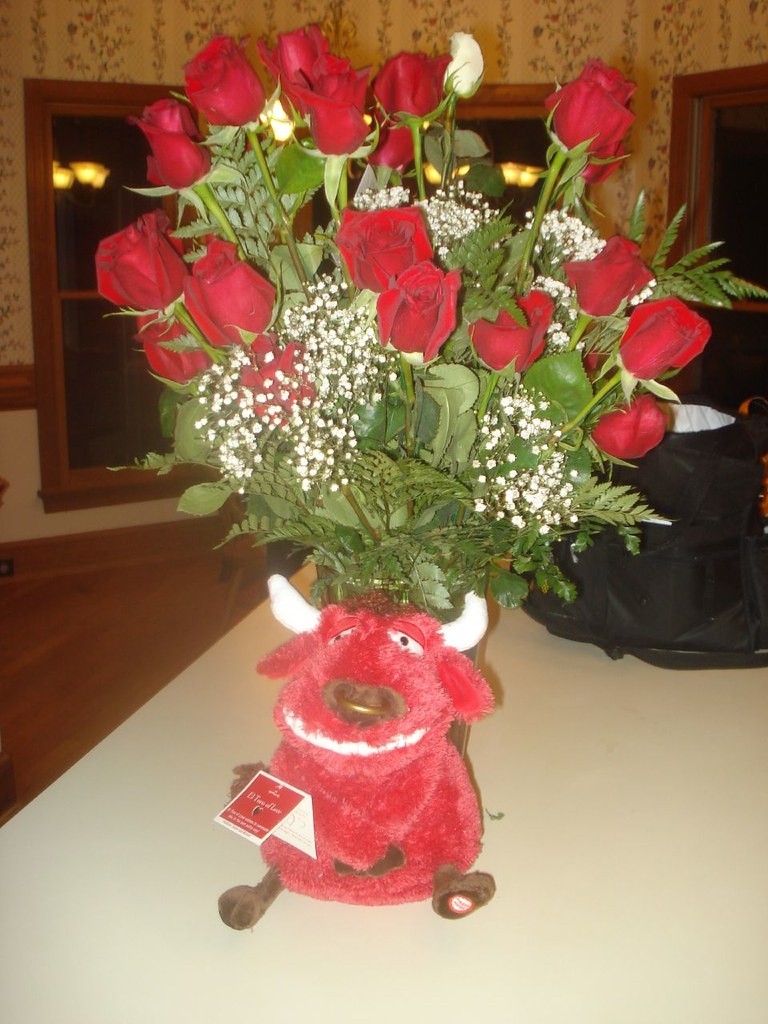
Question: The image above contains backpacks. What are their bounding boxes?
[506,386,768,671]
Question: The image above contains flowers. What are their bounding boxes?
[96,22,768,623]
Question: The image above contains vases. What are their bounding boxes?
[316,550,513,761]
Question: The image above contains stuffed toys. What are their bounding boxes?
[219,574,497,931]
